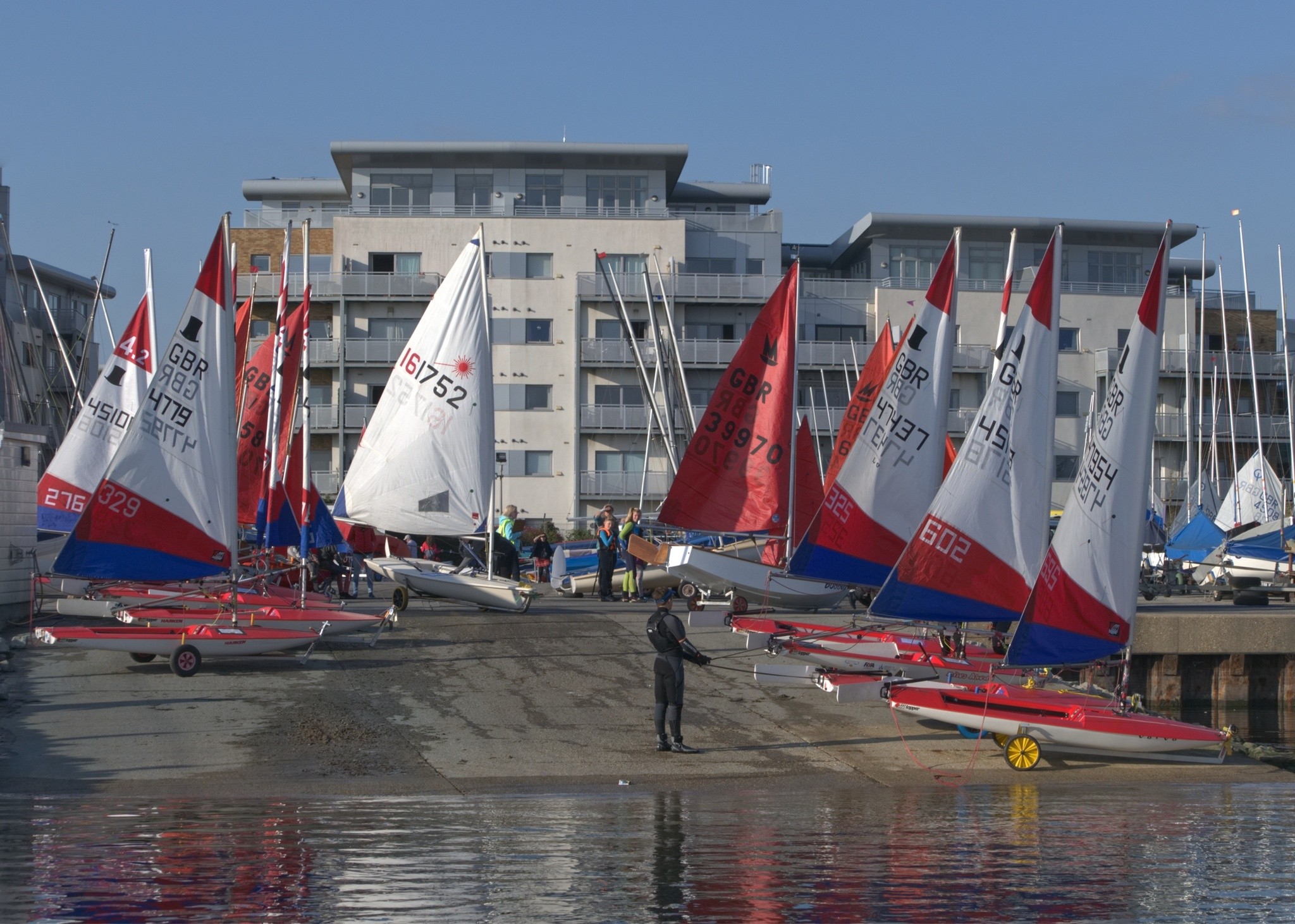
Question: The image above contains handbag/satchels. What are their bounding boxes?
[542,553,550,558]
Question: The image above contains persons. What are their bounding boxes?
[287,546,320,593]
[449,533,516,579]
[647,586,711,753]
[991,621,1013,654]
[618,506,647,603]
[318,545,354,599]
[403,534,417,558]
[530,533,554,582]
[495,504,526,556]
[936,622,962,658]
[419,535,444,561]
[592,504,622,602]
[351,551,375,599]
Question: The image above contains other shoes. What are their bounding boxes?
[340,592,353,599]
[601,596,617,602]
[622,598,630,602]
[309,576,318,582]
[352,593,357,599]
[369,592,375,597]
[632,596,647,602]
[449,571,457,574]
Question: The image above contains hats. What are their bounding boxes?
[654,589,676,602]
[403,535,411,541]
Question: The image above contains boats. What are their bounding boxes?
[204,565,330,592]
[570,534,772,595]
[551,533,751,593]
[33,588,345,618]
[31,570,332,604]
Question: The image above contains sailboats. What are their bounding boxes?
[728,224,1006,664]
[326,222,536,615]
[772,228,1008,665]
[1137,234,1294,594]
[814,222,1116,702]
[113,216,397,632]
[878,218,1226,766]
[656,258,857,608]
[35,212,329,654]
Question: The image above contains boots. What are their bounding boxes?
[657,734,671,751]
[671,736,699,753]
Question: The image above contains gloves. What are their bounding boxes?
[696,654,713,667]
